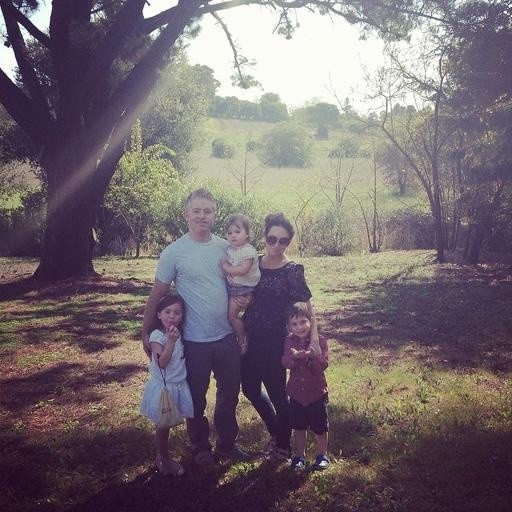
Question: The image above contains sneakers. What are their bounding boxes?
[269,447,291,473]
[238,335,249,355]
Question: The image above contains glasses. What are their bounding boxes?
[266,236,289,244]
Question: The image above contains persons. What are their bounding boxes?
[142,187,250,467]
[242,213,322,462]
[280,301,331,469]
[139,295,194,476]
[220,214,261,356]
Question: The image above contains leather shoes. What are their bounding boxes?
[215,443,251,460]
[291,454,306,470]
[176,464,185,475]
[311,454,330,470]
[194,448,216,466]
